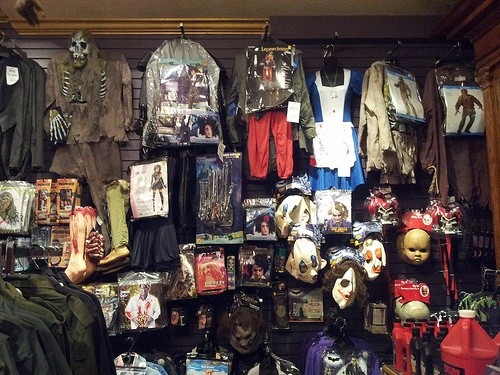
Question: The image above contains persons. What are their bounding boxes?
[452,88,485,134]
[248,253,269,279]
[183,69,204,109]
[150,163,166,212]
[203,124,213,138]
[393,76,418,118]
[253,213,275,239]
[260,50,274,86]
[125,282,162,329]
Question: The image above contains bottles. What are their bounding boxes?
[393,310,500,375]
